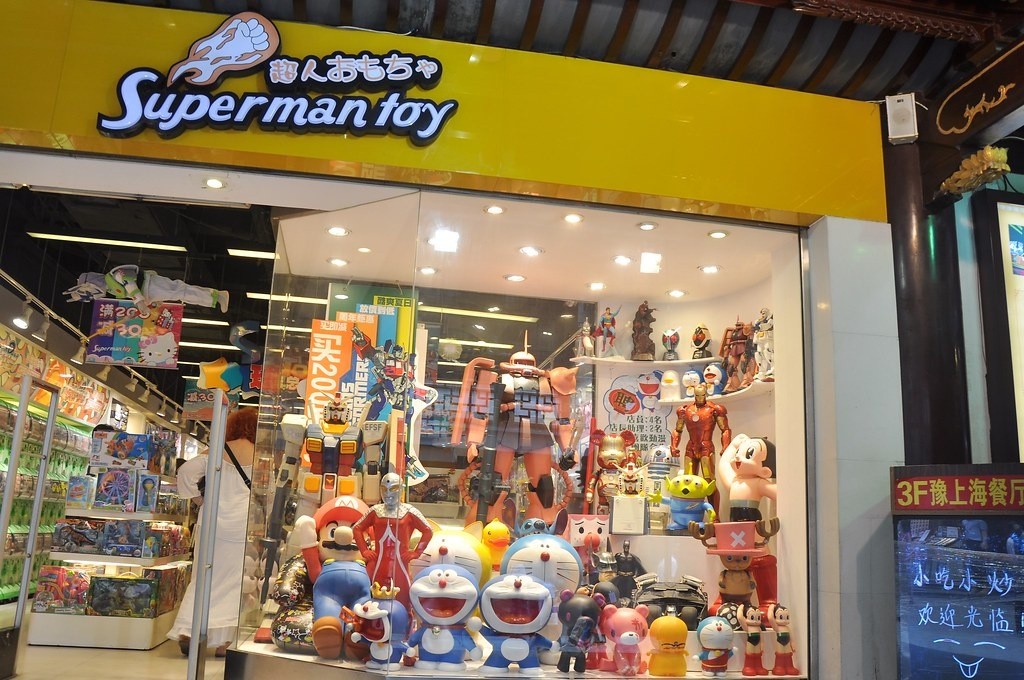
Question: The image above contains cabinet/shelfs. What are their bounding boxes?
[0,400,199,653]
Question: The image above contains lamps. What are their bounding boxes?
[12,295,211,445]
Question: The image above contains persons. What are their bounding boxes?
[178,407,261,657]
[897,516,1024,555]
[265,301,799,677]
